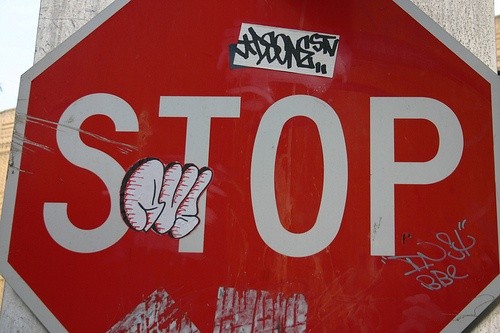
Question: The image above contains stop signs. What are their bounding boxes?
[0,2,499,332]
